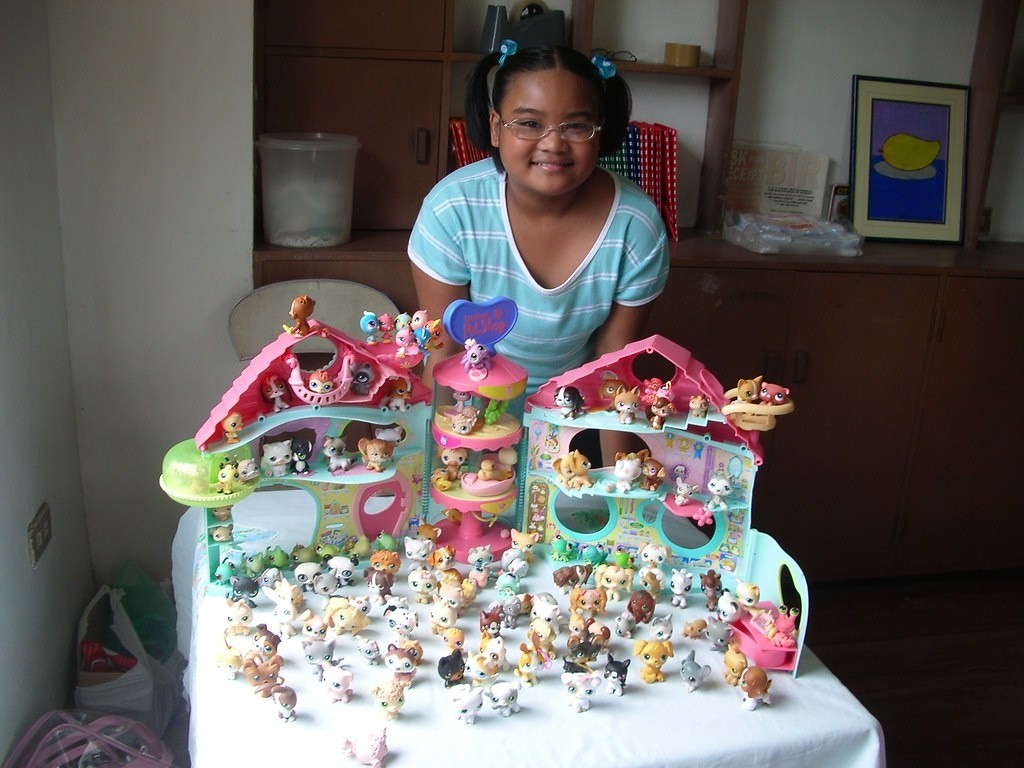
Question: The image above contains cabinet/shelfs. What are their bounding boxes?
[253,0,747,241]
[252,233,1024,580]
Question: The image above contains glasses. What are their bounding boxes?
[500,117,603,142]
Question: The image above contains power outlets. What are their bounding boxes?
[27,503,53,565]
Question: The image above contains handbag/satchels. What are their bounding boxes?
[73,585,174,742]
[2,710,180,768]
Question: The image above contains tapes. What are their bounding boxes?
[664,43,701,67]
[509,0,549,25]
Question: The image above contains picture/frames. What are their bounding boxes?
[848,74,972,247]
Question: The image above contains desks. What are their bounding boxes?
[172,466,887,768]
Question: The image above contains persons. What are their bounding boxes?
[406,41,670,410]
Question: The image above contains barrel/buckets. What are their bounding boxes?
[254,130,359,247]
